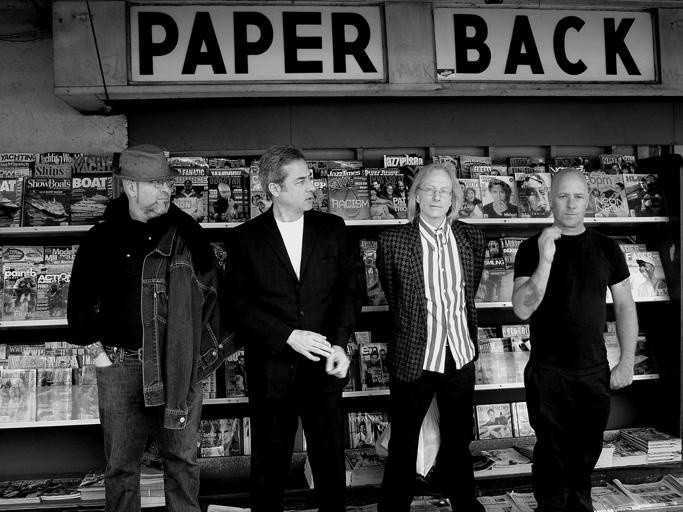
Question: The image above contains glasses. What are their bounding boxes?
[417,185,452,198]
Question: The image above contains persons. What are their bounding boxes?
[511,169,639,512]
[226,147,366,510]
[376,165,485,512]
[66,151,221,512]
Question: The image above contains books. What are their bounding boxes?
[1,236,682,510]
[1,153,665,227]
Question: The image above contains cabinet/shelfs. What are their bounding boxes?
[0,144,683,512]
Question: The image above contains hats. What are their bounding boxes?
[113,143,180,183]
